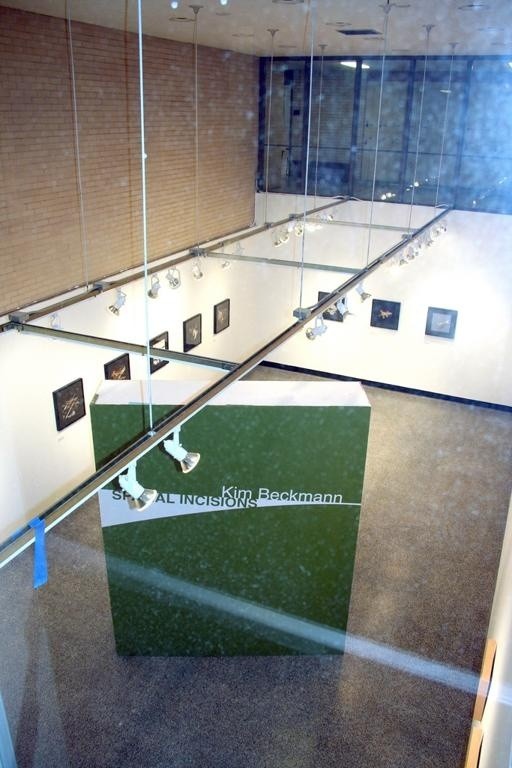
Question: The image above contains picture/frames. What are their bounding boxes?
[424,306,458,340]
[147,331,170,374]
[319,290,341,324]
[181,312,203,354]
[49,378,86,432]
[212,297,231,335]
[104,352,131,380]
[369,298,402,332]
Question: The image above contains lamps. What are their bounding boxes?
[267,223,290,248]
[107,285,126,319]
[50,312,64,339]
[335,294,352,322]
[164,264,183,290]
[146,272,162,299]
[191,254,204,280]
[217,241,248,269]
[355,283,372,303]
[395,213,449,269]
[292,210,333,242]
[305,318,328,343]
[160,426,203,476]
[115,463,160,514]
